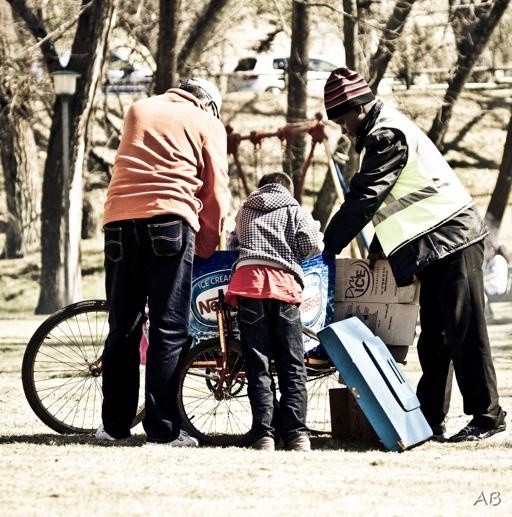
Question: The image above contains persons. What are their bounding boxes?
[95,78,230,446]
[226,173,325,450]
[323,68,506,441]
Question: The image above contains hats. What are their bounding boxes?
[324,68,376,120]
[178,77,223,119]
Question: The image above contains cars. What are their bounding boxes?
[29,36,159,95]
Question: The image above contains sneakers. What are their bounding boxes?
[448,406,507,442]
[95,423,116,442]
[146,429,199,447]
[430,419,446,435]
[282,436,311,452]
[239,436,275,451]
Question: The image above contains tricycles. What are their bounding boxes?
[19,110,369,449]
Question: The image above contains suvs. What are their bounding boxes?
[227,51,396,102]
